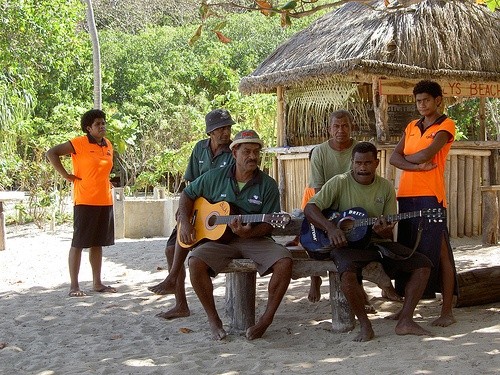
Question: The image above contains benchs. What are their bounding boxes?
[221,256,402,332]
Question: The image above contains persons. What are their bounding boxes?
[300,109,403,315]
[303,142,436,343]
[388,79,460,328]
[147,108,236,319]
[178,130,293,340]
[47,110,116,297]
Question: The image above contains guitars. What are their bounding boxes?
[177,196,291,248]
[299,207,447,252]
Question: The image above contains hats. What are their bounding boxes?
[205,109,236,133]
[229,129,262,150]
